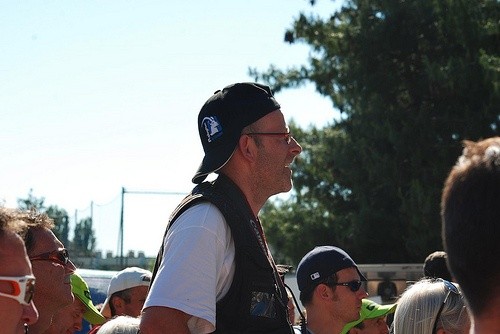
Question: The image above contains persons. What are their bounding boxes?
[392,281,472,334]
[341,299,398,334]
[53,272,104,332]
[296,246,367,334]
[442,137,499,334]
[101,264,152,316]
[0,213,38,332]
[12,201,76,332]
[423,251,449,280]
[94,313,143,333]
[139,82,302,334]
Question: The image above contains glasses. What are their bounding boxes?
[29,248,69,265]
[322,280,361,293]
[431,278,460,334]
[240,132,290,144]
[1,275,37,305]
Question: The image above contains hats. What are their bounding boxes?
[340,299,397,334]
[100,266,153,318]
[192,83,280,183]
[70,274,106,325]
[297,245,366,291]
